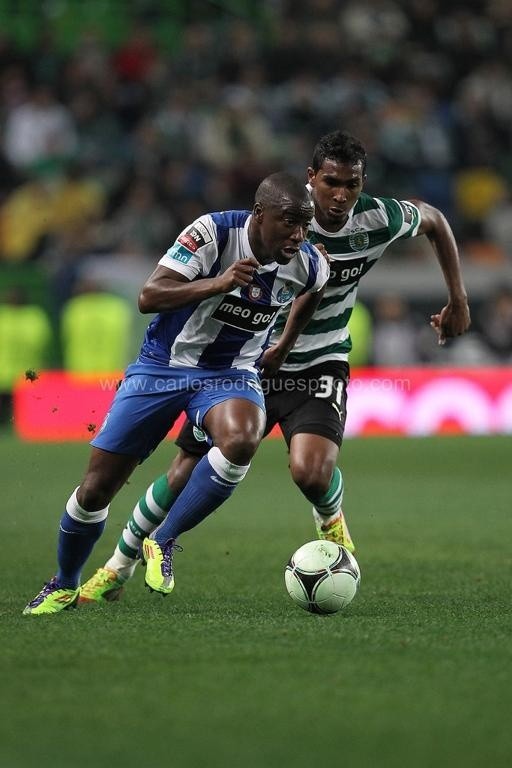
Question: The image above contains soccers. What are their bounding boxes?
[284,539,360,615]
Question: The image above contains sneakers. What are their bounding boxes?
[22,568,125,616]
[142,537,183,597]
[312,507,356,553]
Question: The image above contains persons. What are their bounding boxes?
[22,173,331,615]
[78,132,471,604]
